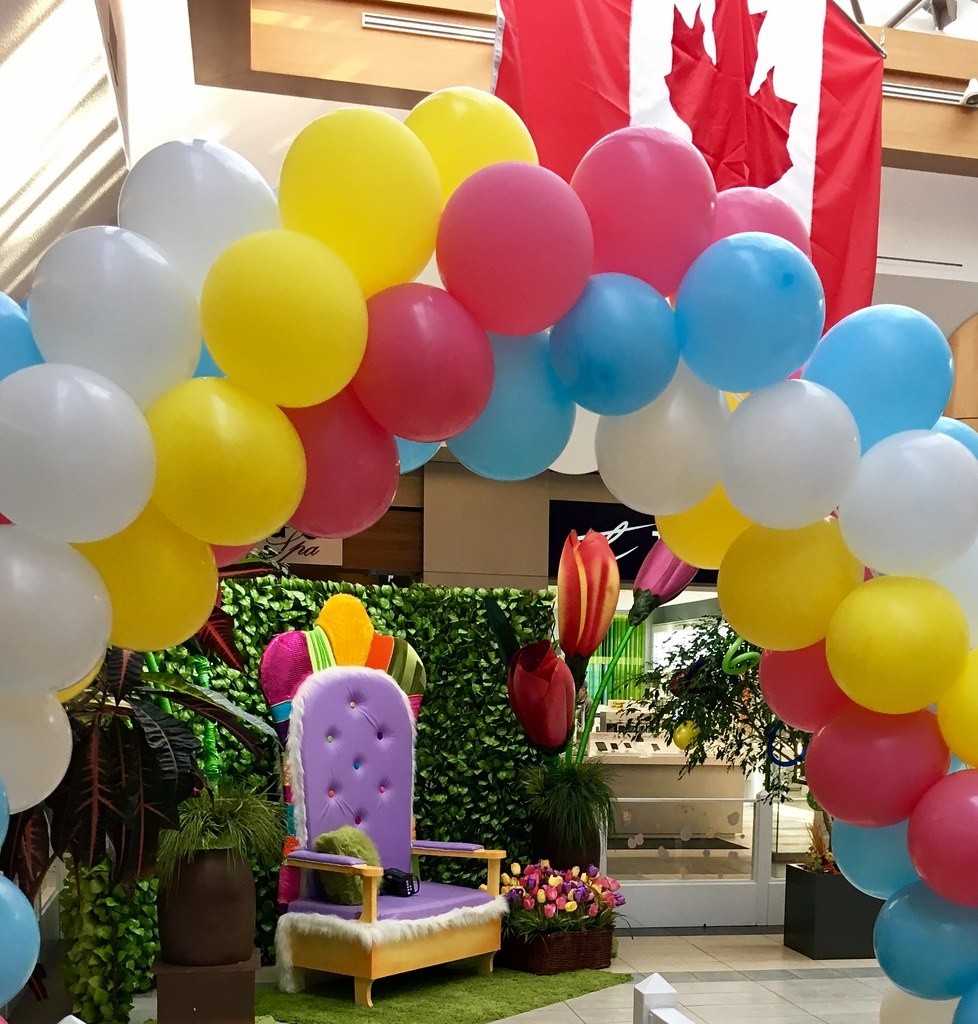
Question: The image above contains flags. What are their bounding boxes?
[490,0,884,339]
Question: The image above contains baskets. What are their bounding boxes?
[504,925,614,976]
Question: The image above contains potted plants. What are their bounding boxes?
[152,781,299,966]
[625,612,886,960]
[527,757,622,867]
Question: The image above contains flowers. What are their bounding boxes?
[482,861,619,934]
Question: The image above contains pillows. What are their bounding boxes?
[314,827,385,905]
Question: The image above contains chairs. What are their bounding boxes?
[259,591,507,1010]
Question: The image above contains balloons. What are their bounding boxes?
[0,86,978,1024]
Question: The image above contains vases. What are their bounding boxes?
[509,925,619,975]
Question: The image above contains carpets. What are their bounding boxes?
[256,968,637,1024]
